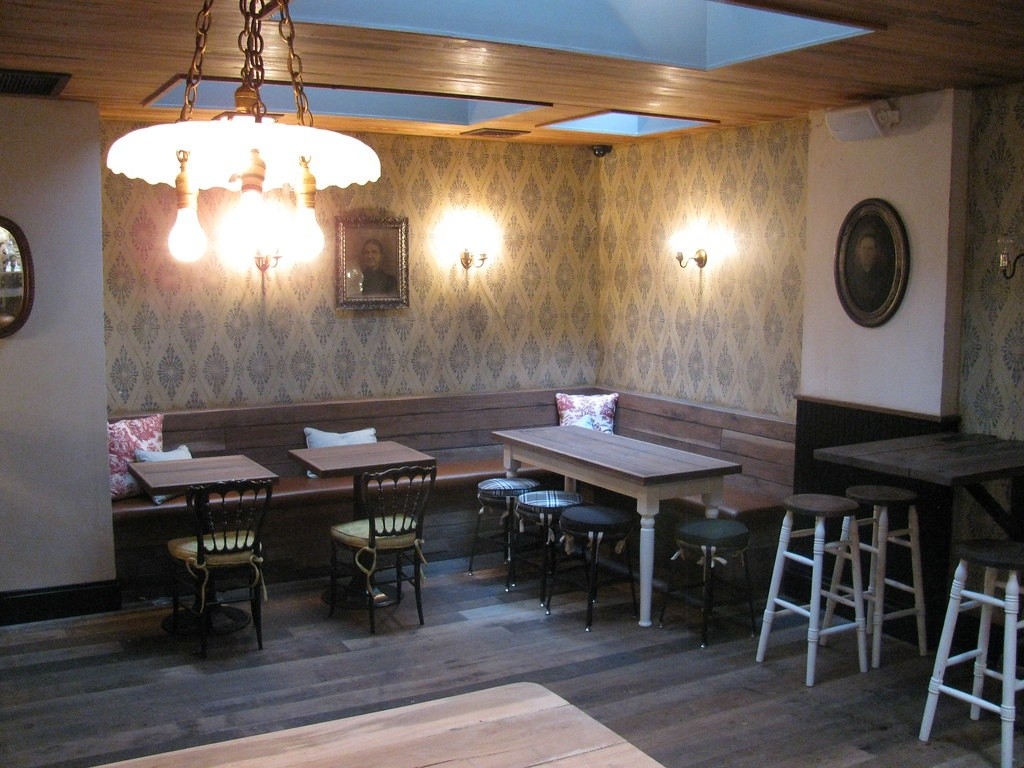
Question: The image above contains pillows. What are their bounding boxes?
[304,426,378,479]
[555,393,618,436]
[107,411,194,506]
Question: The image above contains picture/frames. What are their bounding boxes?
[0,216,35,339]
[832,198,910,328]
[334,217,410,310]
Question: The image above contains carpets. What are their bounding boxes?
[0,509,1024,768]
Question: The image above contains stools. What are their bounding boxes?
[467,478,1024,768]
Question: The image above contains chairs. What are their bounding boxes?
[167,477,278,660]
[330,466,437,636]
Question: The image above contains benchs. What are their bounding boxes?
[112,458,798,609]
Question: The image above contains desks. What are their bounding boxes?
[288,440,437,608]
[491,426,742,628]
[127,455,280,636]
[814,432,1024,547]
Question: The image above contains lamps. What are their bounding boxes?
[461,243,488,268]
[99,0,382,268]
[669,233,706,269]
[994,233,1024,279]
[250,238,283,272]
